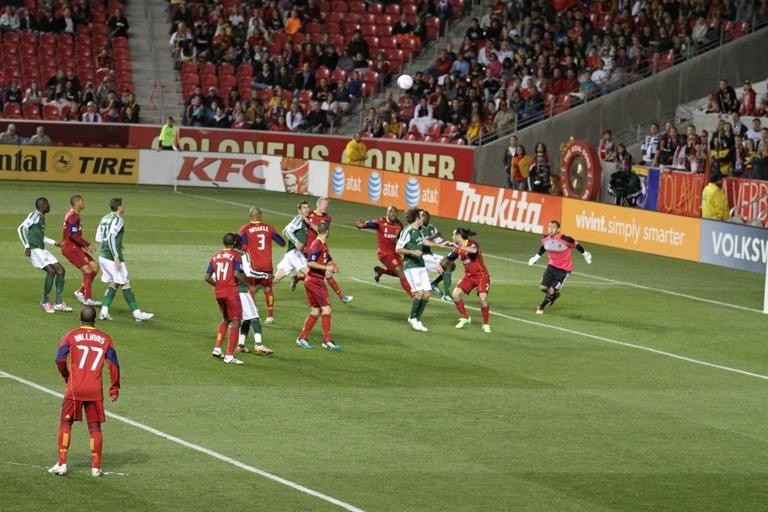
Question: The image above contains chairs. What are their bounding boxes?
[360,125,465,145]
[720,21,733,31]
[732,21,750,38]
[658,53,674,71]
[544,93,555,114]
[0,0,134,123]
[586,13,623,32]
[181,0,462,102]
[553,94,570,115]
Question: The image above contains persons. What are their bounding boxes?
[1,70,140,123]
[96,45,115,82]
[205,197,353,365]
[47,306,121,477]
[608,158,642,207]
[1,124,53,145]
[503,136,562,196]
[597,123,768,181]
[354,205,491,334]
[106,8,129,42]
[164,1,392,134]
[16,196,154,322]
[346,132,368,167]
[701,171,729,220]
[156,115,176,152]
[374,1,768,149]
[1,1,93,36]
[527,221,592,315]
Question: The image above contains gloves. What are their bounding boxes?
[528,254,541,266]
[582,250,591,265]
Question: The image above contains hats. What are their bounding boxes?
[710,172,728,182]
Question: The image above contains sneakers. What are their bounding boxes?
[91,468,104,476]
[132,309,154,320]
[264,316,273,324]
[374,266,381,282]
[321,341,343,352]
[40,289,102,313]
[536,305,543,315]
[296,337,312,349]
[342,295,354,304]
[408,316,423,330]
[290,274,297,293]
[417,321,427,332]
[431,282,491,333]
[48,462,67,476]
[212,342,274,364]
[98,312,113,320]
